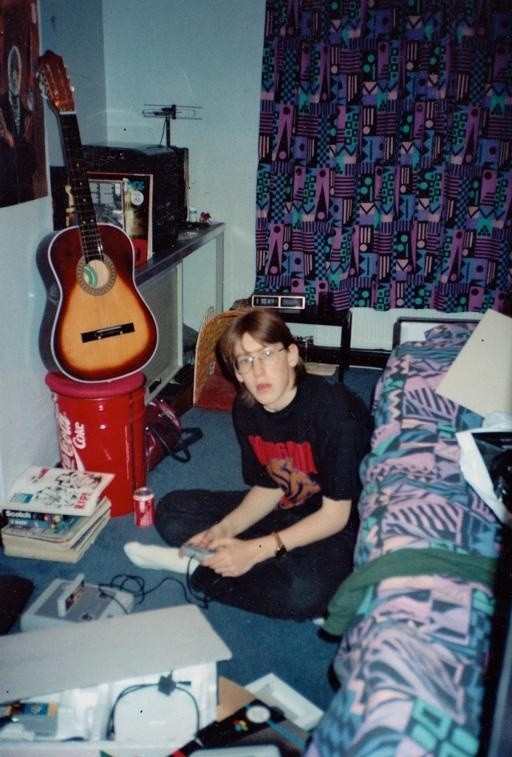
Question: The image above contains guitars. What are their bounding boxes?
[34,49,159,385]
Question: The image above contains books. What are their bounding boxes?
[2,467,116,565]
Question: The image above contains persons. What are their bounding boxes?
[120,308,374,620]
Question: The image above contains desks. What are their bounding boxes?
[135,221,225,406]
[228,300,352,382]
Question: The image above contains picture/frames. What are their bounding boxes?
[0,0,48,208]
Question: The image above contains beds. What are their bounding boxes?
[302,316,512,756]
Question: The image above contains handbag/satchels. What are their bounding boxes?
[142,399,203,474]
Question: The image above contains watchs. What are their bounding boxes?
[269,531,290,562]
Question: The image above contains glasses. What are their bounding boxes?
[234,346,285,375]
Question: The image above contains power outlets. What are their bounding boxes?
[143,104,205,120]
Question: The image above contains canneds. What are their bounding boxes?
[132,488,158,530]
